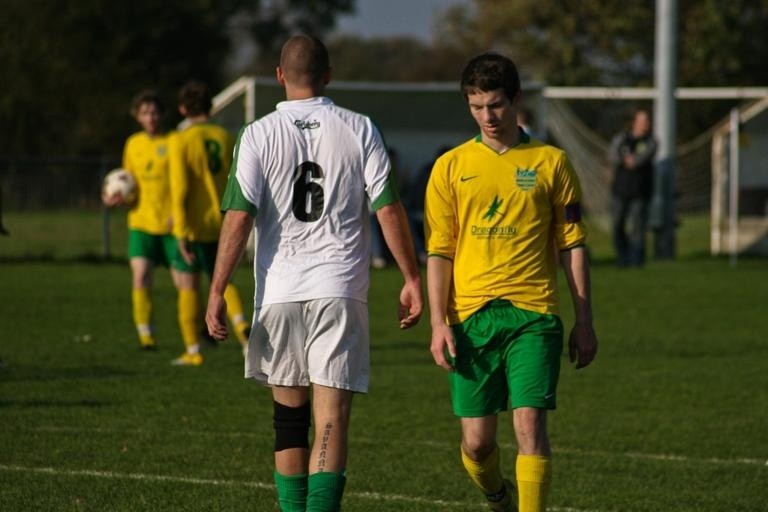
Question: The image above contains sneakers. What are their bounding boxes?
[170,351,202,366]
[489,478,515,511]
[137,345,157,353]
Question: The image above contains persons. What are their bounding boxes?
[516,107,544,141]
[411,144,456,251]
[604,109,659,268]
[203,34,425,512]
[367,147,428,270]
[421,52,599,512]
[101,87,216,352]
[167,79,253,367]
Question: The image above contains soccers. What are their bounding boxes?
[104,169,139,206]
